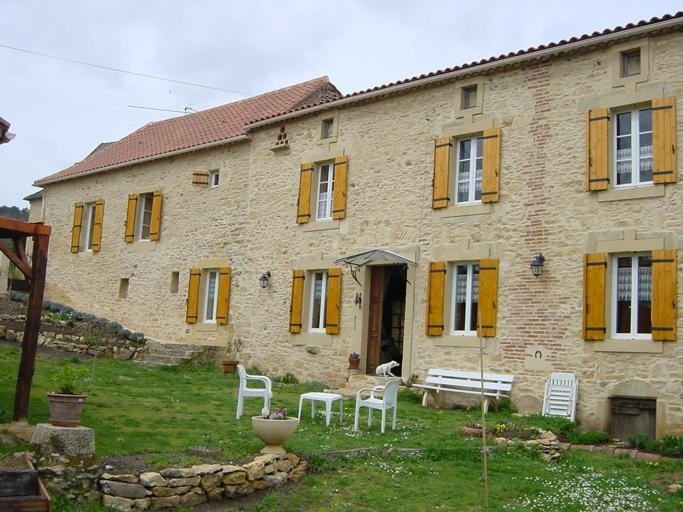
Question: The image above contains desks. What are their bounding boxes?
[296,391,343,427]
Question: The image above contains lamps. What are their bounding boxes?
[530,252,545,279]
[257,269,271,289]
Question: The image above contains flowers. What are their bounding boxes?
[348,350,359,358]
[260,403,289,419]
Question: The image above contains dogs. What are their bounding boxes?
[376,360,400,377]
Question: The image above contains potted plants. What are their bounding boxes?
[221,336,242,373]
[45,359,88,427]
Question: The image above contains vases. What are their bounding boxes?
[250,414,298,454]
[348,359,360,369]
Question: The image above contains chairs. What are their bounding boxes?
[353,378,399,433]
[541,371,578,424]
[234,363,273,420]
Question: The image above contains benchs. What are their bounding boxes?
[410,367,513,414]
